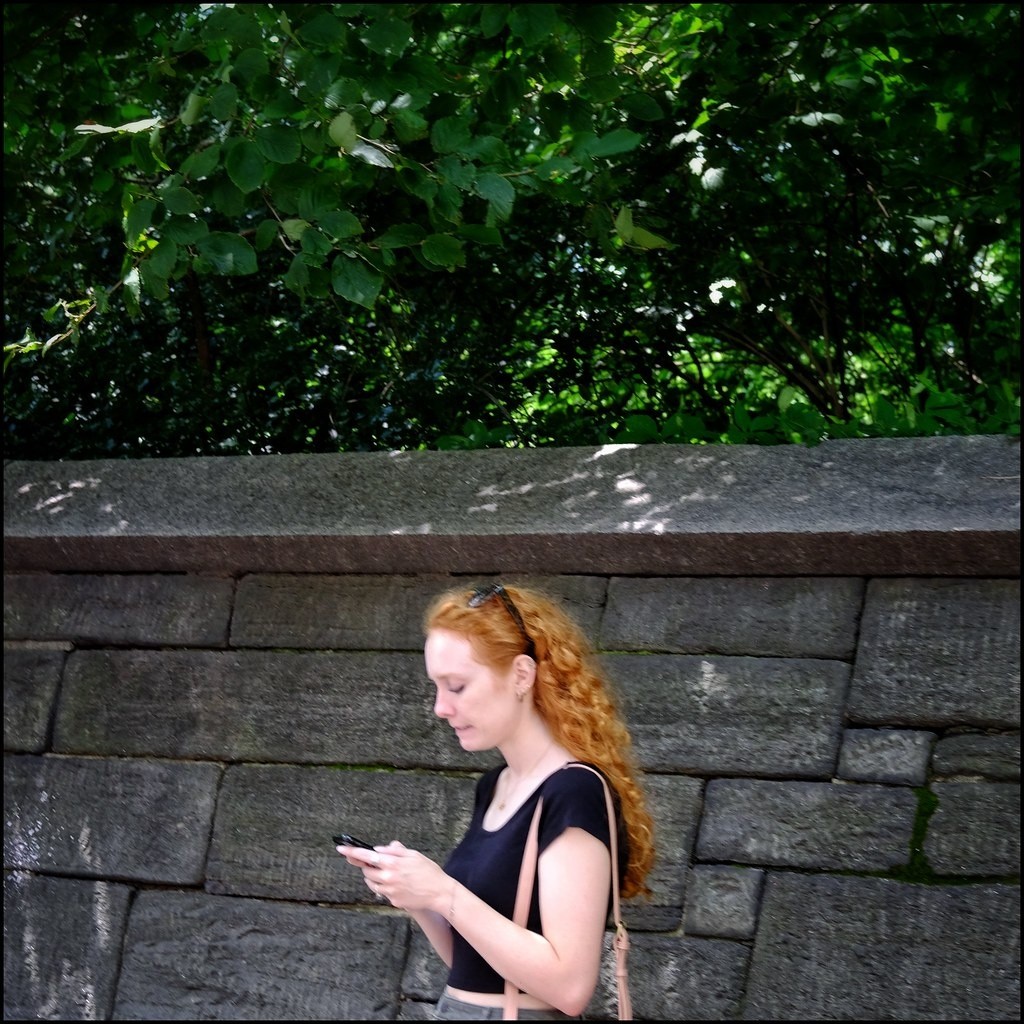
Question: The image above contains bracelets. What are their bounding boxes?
[446,881,458,927]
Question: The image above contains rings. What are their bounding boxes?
[374,884,382,900]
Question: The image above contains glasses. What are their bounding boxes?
[468,582,527,636]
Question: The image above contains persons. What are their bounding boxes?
[336,585,656,1021]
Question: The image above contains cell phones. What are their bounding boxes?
[331,833,378,868]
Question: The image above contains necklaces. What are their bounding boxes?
[499,741,554,811]
